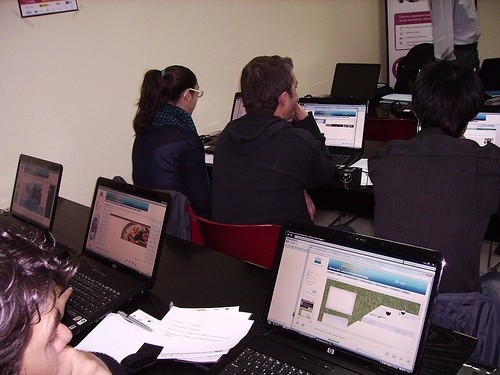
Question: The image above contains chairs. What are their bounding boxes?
[365,118,417,142]
[114,176,285,269]
[435,294,500,369]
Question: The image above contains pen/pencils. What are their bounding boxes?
[117,310,153,332]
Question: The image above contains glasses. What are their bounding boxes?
[183,88,204,98]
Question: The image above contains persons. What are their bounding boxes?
[212,55,345,227]
[430,0,481,78]
[368,60,500,369]
[0,224,114,375]
[132,65,214,219]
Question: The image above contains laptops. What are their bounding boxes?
[0,154,64,239]
[54,177,172,340]
[416,110,500,148]
[330,62,382,104]
[207,220,445,375]
[297,97,369,177]
[201,91,247,155]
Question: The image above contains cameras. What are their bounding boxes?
[334,164,363,185]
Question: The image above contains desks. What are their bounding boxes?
[49,196,478,375]
